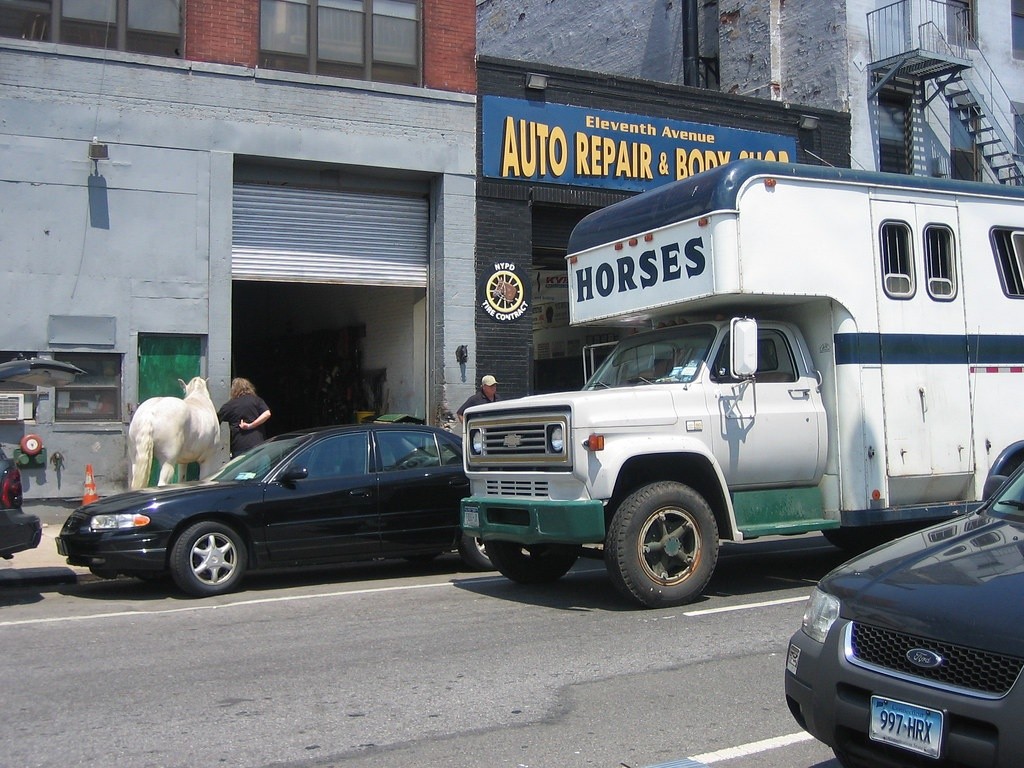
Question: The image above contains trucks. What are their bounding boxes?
[461,158,1024,608]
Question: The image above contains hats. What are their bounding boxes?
[482,375,499,387]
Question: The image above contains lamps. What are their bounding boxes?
[88,136,109,161]
[525,71,549,90]
[798,115,820,129]
[456,345,468,363]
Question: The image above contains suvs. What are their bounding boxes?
[0,443,42,560]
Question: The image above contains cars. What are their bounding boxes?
[785,460,1024,768]
[55,424,472,598]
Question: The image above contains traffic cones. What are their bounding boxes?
[81,464,99,505]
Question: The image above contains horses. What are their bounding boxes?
[125,376,221,491]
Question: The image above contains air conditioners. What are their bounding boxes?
[0,393,24,421]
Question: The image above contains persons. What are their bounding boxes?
[457,374,503,425]
[216,377,271,461]
